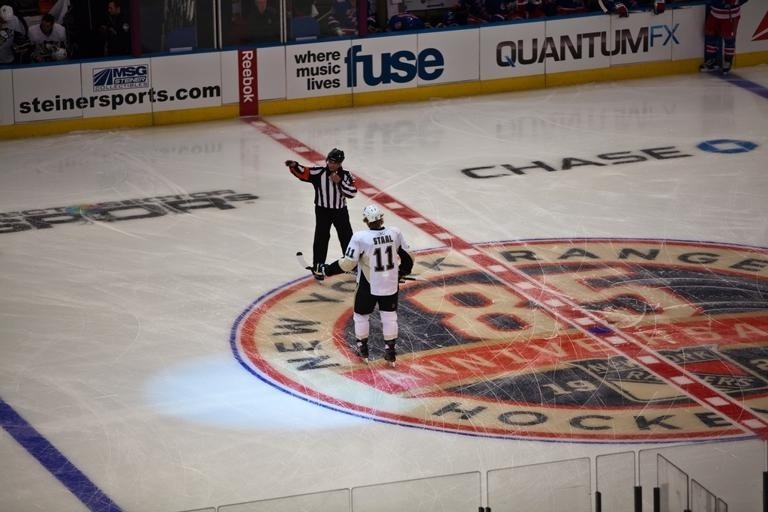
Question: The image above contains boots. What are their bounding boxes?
[353,338,369,358]
[384,340,397,361]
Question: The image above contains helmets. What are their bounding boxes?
[328,148,344,163]
[363,203,384,224]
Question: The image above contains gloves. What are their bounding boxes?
[313,263,326,276]
[398,270,406,280]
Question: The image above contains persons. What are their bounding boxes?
[311,204,415,362]
[390,1,665,32]
[164,1,194,49]
[248,1,382,42]
[1,2,132,67]
[700,2,747,74]
[285,148,359,280]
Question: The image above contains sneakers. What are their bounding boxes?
[315,275,324,280]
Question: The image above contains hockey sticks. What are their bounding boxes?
[296,252,416,280]
